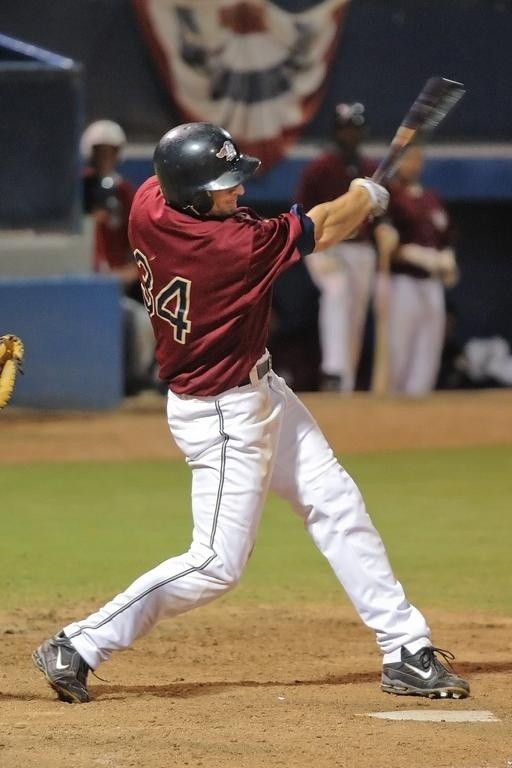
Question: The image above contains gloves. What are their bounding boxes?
[348,176,390,225]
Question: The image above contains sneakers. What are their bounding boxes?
[380,646,470,699]
[31,629,90,703]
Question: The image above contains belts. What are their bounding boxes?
[238,355,272,387]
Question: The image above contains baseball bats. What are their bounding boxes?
[372,253,389,392]
[343,75,466,241]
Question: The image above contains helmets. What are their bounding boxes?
[79,119,127,160]
[152,122,262,215]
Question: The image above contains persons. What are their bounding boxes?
[32,122,470,704]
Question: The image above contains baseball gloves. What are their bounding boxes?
[0,334,25,408]
